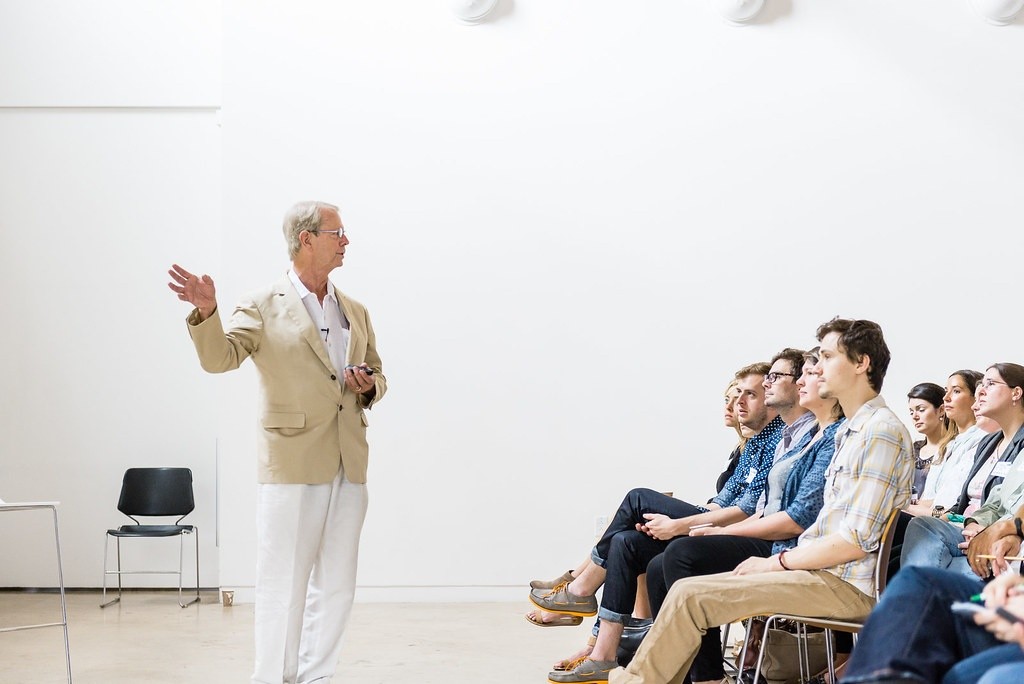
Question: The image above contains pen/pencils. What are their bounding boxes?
[975,555,1024,561]
[995,607,1024,626]
[969,583,1024,602]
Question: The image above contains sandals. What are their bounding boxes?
[525,608,583,626]
[552,636,597,670]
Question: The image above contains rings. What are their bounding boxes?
[975,560,980,562]
[354,386,362,392]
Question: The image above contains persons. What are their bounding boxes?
[169,202,388,684]
[835,363,1024,684]
[526,315,914,684]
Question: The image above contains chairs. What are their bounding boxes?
[721,509,901,684]
[100,467,199,608]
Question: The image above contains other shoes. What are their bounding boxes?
[530,569,576,589]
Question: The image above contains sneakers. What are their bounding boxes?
[548,656,619,683]
[529,581,598,617]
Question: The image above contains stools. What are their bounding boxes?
[0,497,73,684]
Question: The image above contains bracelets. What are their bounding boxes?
[1014,518,1023,538]
[778,550,792,570]
[932,505,946,517]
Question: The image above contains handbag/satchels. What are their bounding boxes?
[593,612,655,668]
[735,618,817,669]
[761,628,837,684]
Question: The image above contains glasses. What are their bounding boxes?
[975,378,1017,389]
[763,372,800,383]
[307,228,345,238]
